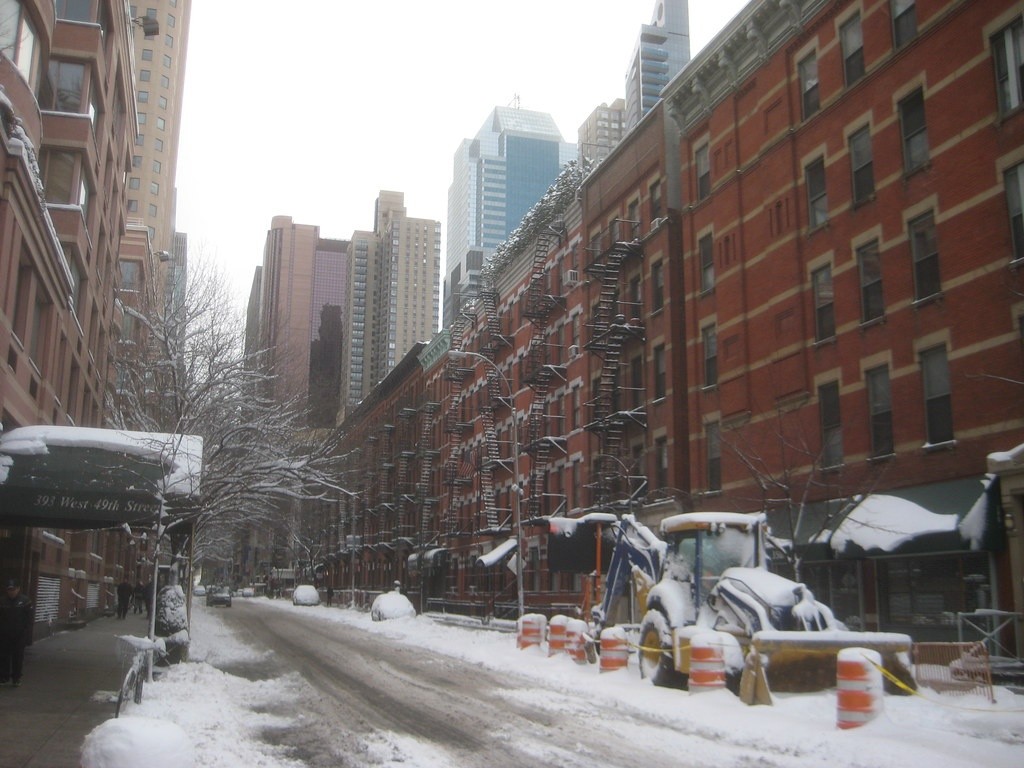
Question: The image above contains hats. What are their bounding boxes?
[6,578,19,590]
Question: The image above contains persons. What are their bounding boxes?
[1,579,34,687]
[327,586,334,606]
[116,574,153,620]
[393,580,401,595]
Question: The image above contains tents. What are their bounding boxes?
[0,422,206,637]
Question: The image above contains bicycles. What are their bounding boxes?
[110,633,170,719]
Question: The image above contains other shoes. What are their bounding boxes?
[12,678,21,687]
[117,616,121,619]
[123,616,125,619]
[0,678,10,685]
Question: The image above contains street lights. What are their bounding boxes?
[448,348,526,618]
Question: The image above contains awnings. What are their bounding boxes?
[761,475,1006,564]
[475,538,518,567]
[407,547,448,568]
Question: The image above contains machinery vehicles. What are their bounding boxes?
[590,506,919,705]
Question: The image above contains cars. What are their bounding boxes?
[193,584,215,598]
[204,587,230,607]
